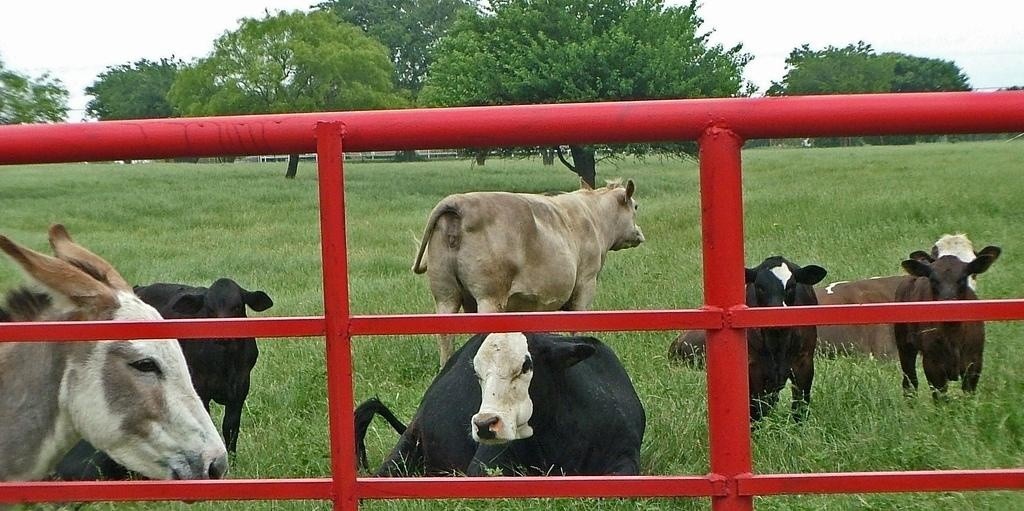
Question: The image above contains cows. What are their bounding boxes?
[894,254,994,411]
[408,175,646,376]
[354,332,647,507]
[667,229,1002,372]
[744,255,828,432]
[132,277,275,466]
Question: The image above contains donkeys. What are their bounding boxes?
[0,221,232,511]
